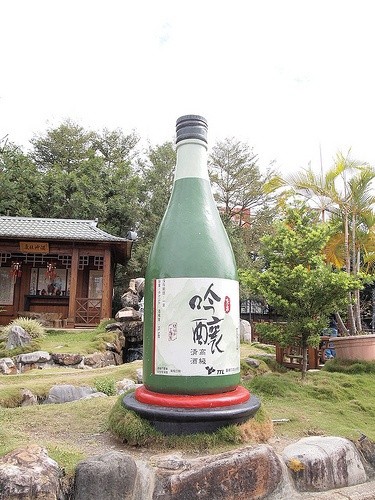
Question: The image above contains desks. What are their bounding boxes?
[24,295,69,312]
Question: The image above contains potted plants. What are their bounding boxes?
[263,144,375,362]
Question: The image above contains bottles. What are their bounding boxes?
[141,116,242,393]
